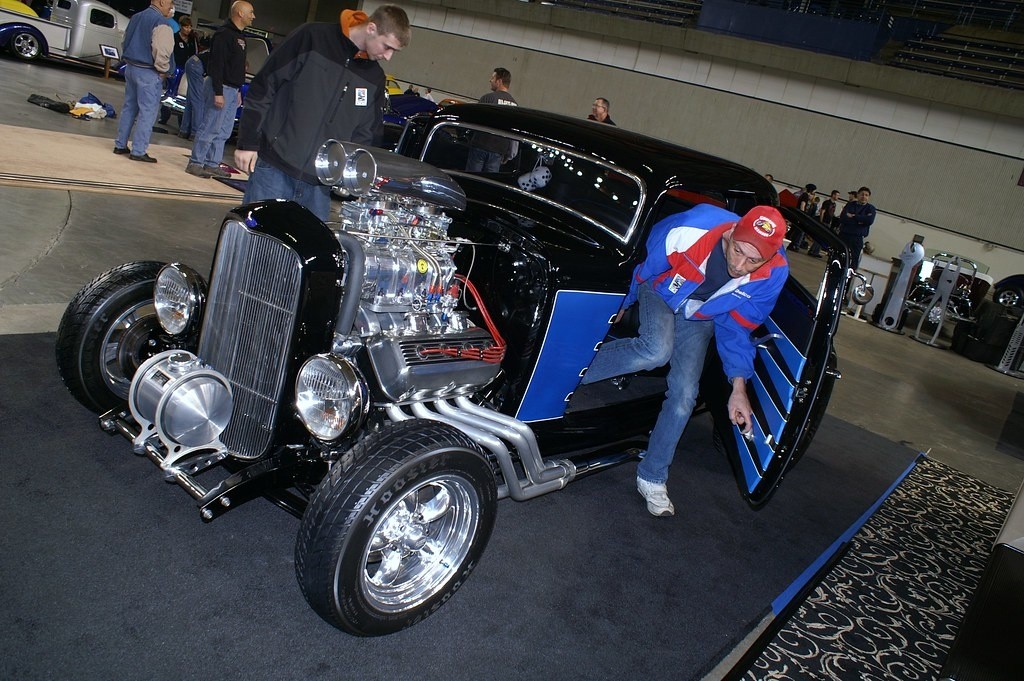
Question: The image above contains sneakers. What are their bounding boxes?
[203,163,231,178]
[185,163,211,179]
[636,475,674,517]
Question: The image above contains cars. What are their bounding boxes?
[52,99,875,639]
[992,273,1024,310]
[159,21,278,139]
[382,73,469,141]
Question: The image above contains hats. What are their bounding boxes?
[734,206,787,261]
[848,191,858,196]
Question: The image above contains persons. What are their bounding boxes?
[579,205,789,516]
[839,186,876,270]
[765,174,773,183]
[158,16,209,139]
[404,85,420,97]
[466,67,519,172]
[234,4,412,222]
[113,0,174,163]
[185,0,256,178]
[587,98,616,126]
[787,183,858,258]
[423,88,433,102]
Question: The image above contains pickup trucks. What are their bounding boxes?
[0,0,133,77]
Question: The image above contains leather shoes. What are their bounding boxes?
[113,146,131,155]
[128,152,157,163]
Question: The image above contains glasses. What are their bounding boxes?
[592,104,605,108]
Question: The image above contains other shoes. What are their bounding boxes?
[178,132,190,139]
[157,119,167,124]
[808,251,823,259]
[189,135,195,141]
[787,245,800,253]
